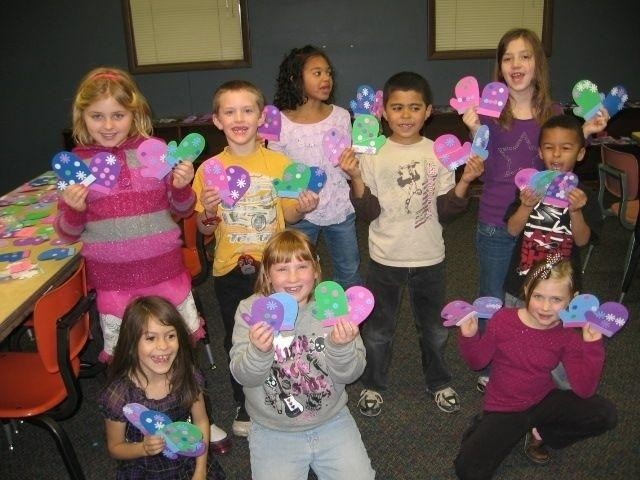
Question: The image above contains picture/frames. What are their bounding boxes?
[120,1,254,76]
[427,0,553,60]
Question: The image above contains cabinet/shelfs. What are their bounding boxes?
[60,102,638,185]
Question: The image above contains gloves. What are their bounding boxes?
[433,134,472,172]
[51,151,97,191]
[476,82,509,119]
[0,176,59,237]
[350,85,376,118]
[268,292,298,331]
[559,294,599,327]
[600,84,628,118]
[200,158,230,200]
[164,133,206,167]
[242,298,284,330]
[37,247,75,262]
[136,139,173,180]
[449,76,480,115]
[13,233,49,246]
[307,167,327,193]
[220,165,250,208]
[257,105,281,141]
[472,297,503,319]
[373,90,384,120]
[271,163,310,200]
[470,125,490,161]
[123,403,206,461]
[323,127,351,167]
[88,152,121,195]
[50,238,81,247]
[572,80,603,122]
[351,114,386,155]
[308,281,348,328]
[584,301,628,337]
[345,286,374,325]
[441,301,478,326]
[0,249,31,262]
[515,167,579,209]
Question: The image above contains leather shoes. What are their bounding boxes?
[211,434,232,454]
[524,430,550,463]
[232,419,252,438]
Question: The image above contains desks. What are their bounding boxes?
[0,169,83,345]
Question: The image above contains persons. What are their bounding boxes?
[502,116,604,310]
[463,29,611,395]
[256,44,363,288]
[228,230,376,476]
[455,253,617,475]
[54,67,232,453]
[338,72,484,416]
[191,81,319,437]
[105,296,223,476]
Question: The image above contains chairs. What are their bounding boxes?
[581,143,639,305]
[7,282,93,353]
[0,256,105,480]
[180,213,216,369]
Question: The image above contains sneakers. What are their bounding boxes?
[426,387,461,412]
[358,389,383,416]
[477,377,489,393]
